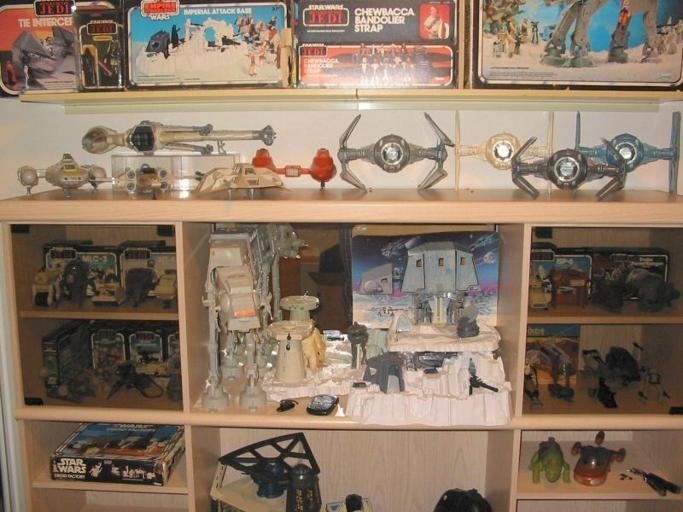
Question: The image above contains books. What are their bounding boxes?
[48,423,184,489]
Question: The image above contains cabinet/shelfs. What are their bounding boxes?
[1,189,683,512]
